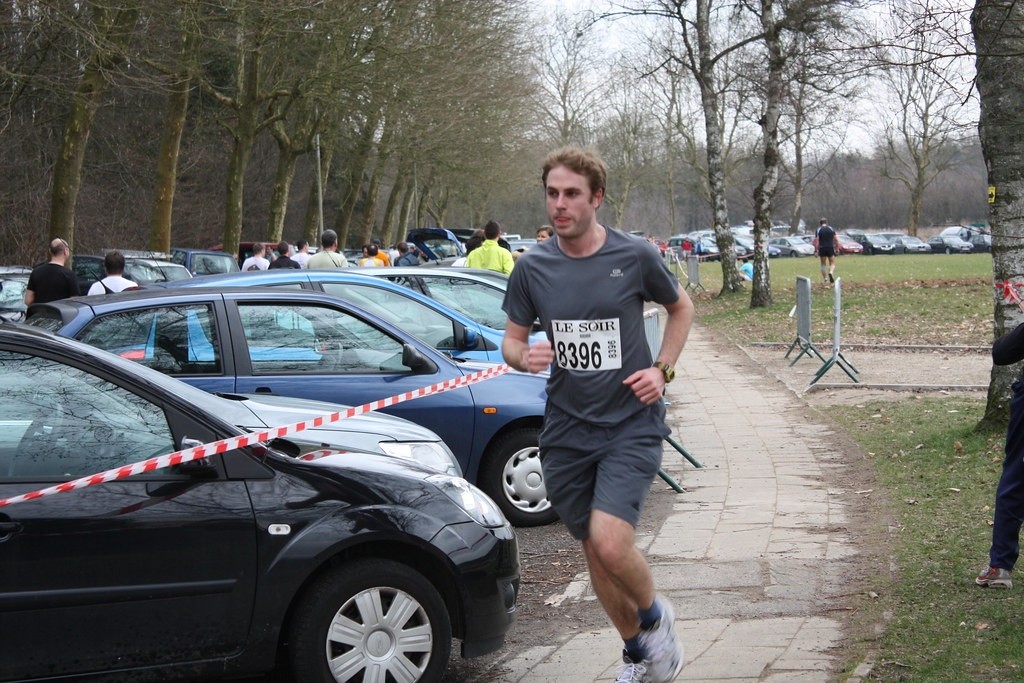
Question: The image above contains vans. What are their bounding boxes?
[939,226,980,241]
[168,248,240,278]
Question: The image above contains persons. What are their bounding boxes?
[266,245,277,263]
[695,237,706,263]
[974,323,1024,589]
[452,218,555,276]
[241,243,270,272]
[815,217,839,283]
[357,241,456,268]
[290,239,312,269]
[87,251,139,296]
[735,258,753,281]
[268,241,301,270]
[24,238,81,306]
[305,229,348,269]
[682,237,692,261]
[501,145,694,683]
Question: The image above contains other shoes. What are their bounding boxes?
[828,272,834,283]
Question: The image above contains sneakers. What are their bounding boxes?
[639,598,684,683]
[976,567,1013,589]
[614,649,649,683]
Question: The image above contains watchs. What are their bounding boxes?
[652,362,675,383]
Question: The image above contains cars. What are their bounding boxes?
[344,266,549,342]
[0,368,463,480]
[811,235,864,255]
[925,236,974,254]
[768,237,816,257]
[655,236,746,262]
[1,265,34,323]
[802,235,816,242]
[32,254,172,297]
[21,288,556,524]
[732,234,781,258]
[406,227,512,261]
[101,269,555,369]
[968,234,993,253]
[1,318,520,683]
[846,229,896,255]
[889,236,931,254]
[134,259,194,282]
